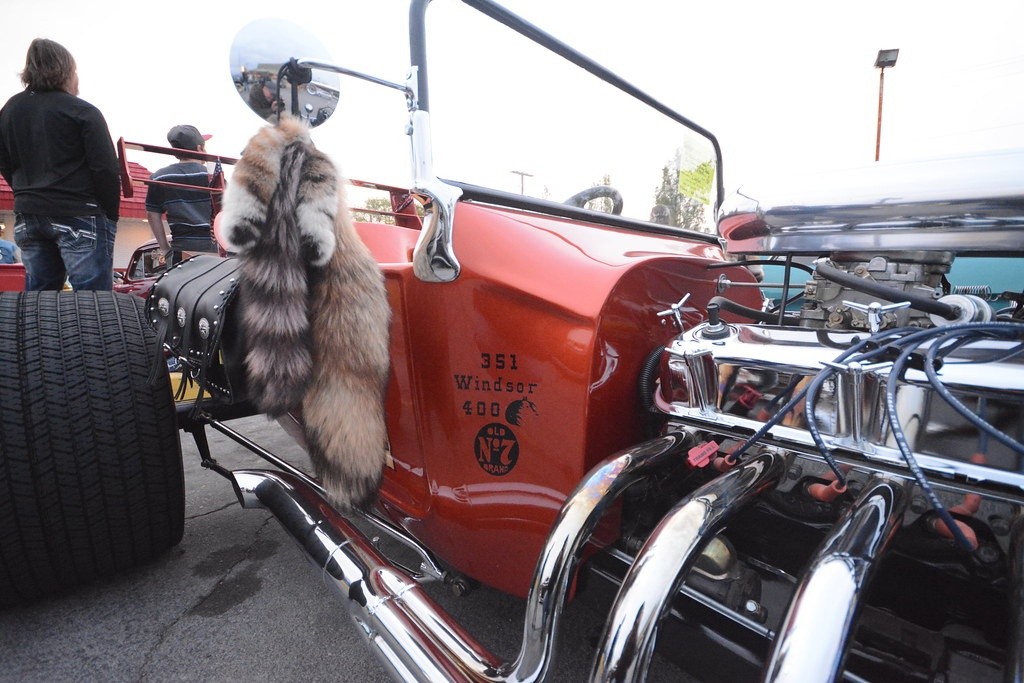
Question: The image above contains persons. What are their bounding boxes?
[0,226,21,264]
[145,125,217,261]
[0,37,121,292]
[241,67,285,119]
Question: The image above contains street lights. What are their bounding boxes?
[872,48,901,162]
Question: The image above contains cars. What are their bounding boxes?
[110,234,174,300]
[0,1,1021,683]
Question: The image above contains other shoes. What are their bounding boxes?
[165,356,184,373]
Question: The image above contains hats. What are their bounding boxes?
[167,124,212,150]
